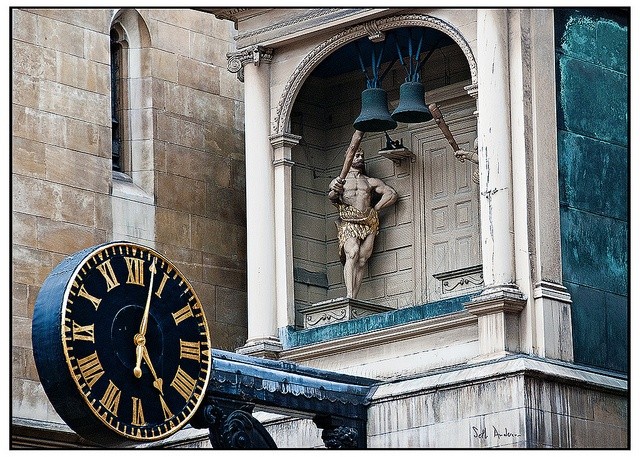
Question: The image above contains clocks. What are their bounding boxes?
[32,241,212,448]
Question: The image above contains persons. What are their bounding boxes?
[454,138,479,263]
[327,147,399,300]
